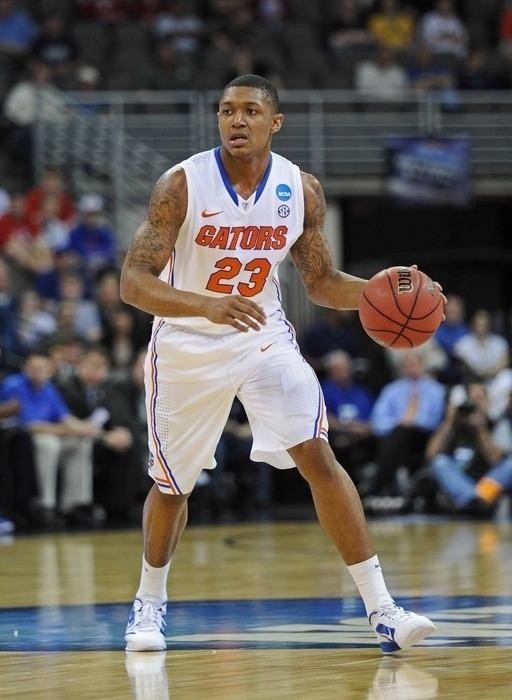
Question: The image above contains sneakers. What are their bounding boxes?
[124,595,168,652]
[369,603,436,654]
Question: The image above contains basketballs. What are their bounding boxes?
[359,265,444,349]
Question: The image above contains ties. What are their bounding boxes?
[402,381,418,426]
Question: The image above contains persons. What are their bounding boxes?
[1,1,512,197]
[120,75,447,656]
[122,648,440,699]
[1,167,511,519]
